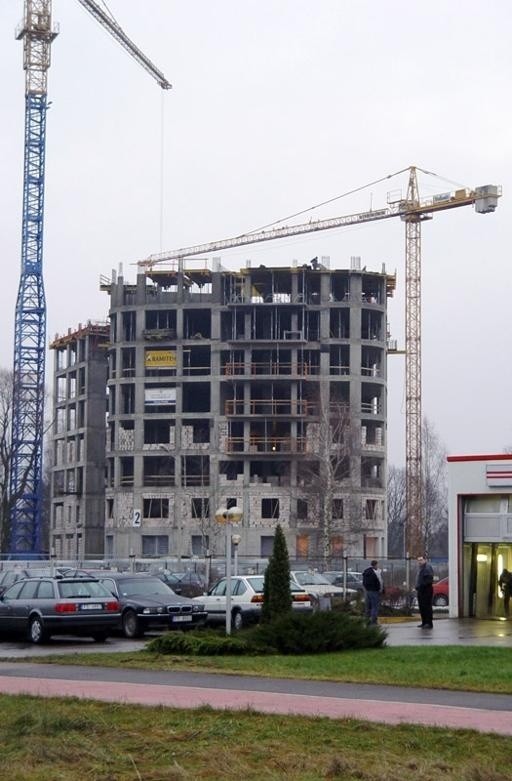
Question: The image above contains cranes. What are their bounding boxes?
[10,0,172,560]
[138,166,504,560]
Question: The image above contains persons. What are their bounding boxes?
[415,556,434,628]
[310,256,318,270]
[499,569,512,617]
[361,561,385,627]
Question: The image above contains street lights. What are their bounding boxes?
[231,535,241,576]
[213,505,243,637]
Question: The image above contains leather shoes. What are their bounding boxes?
[418,624,433,628]
